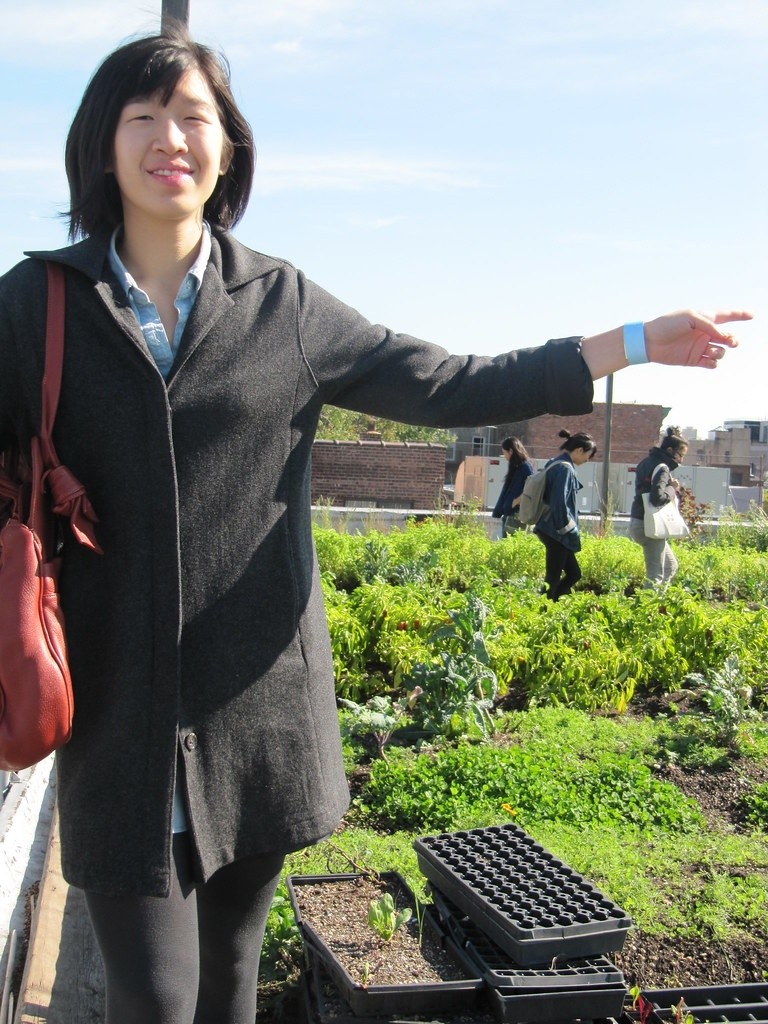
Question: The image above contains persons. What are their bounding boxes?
[0,34,754,1024]
[628,427,689,596]
[532,428,597,603]
[492,437,534,537]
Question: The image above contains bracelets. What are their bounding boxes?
[622,320,650,365]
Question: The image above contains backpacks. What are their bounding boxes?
[520,460,574,525]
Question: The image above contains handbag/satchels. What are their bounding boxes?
[642,463,690,539]
[505,515,529,534]
[0,257,104,771]
